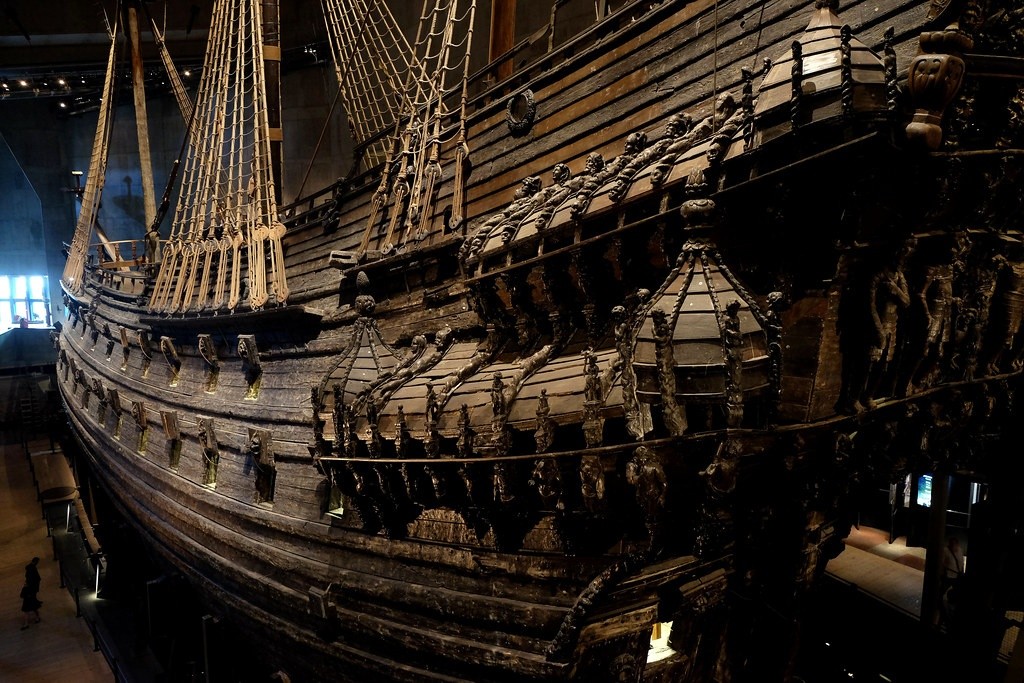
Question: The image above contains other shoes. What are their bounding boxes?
[20,625,29,631]
[34,618,41,624]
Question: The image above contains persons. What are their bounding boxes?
[20,557,43,630]
[311,83,1024,560]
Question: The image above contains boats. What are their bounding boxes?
[50,0,1024,683]
